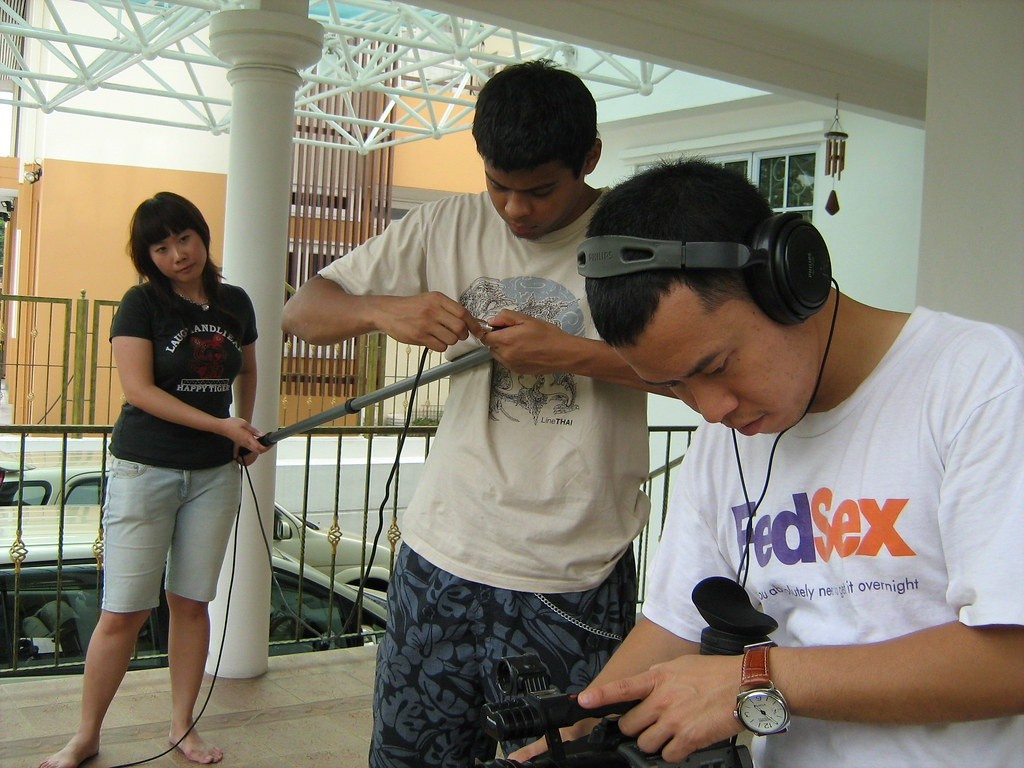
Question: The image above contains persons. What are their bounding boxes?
[505,159,1024,768]
[39,191,259,768]
[279,61,678,768]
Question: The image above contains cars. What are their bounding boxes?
[1,464,398,597]
[0,503,386,680]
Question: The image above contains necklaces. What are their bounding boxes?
[174,287,210,311]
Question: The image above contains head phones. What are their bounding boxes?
[575,210,833,327]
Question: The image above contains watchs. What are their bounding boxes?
[733,639,791,736]
